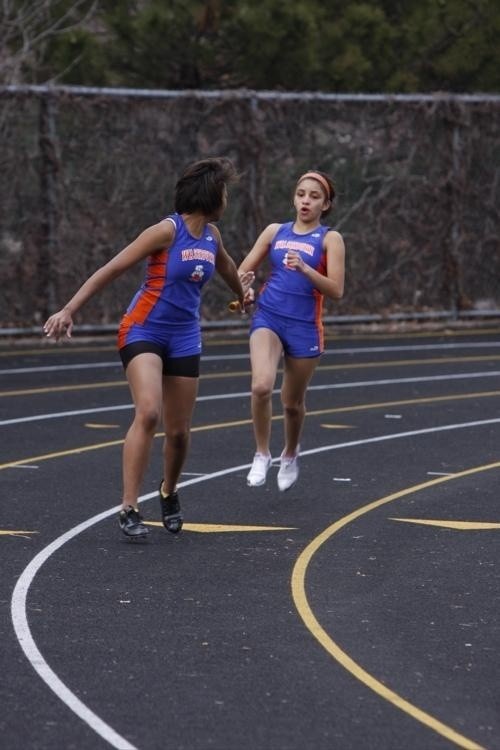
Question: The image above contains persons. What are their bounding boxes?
[237,169,345,492]
[43,160,245,540]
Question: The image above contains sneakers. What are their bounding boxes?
[276,443,301,493]
[118,504,150,538]
[159,478,184,534]
[246,451,273,488]
